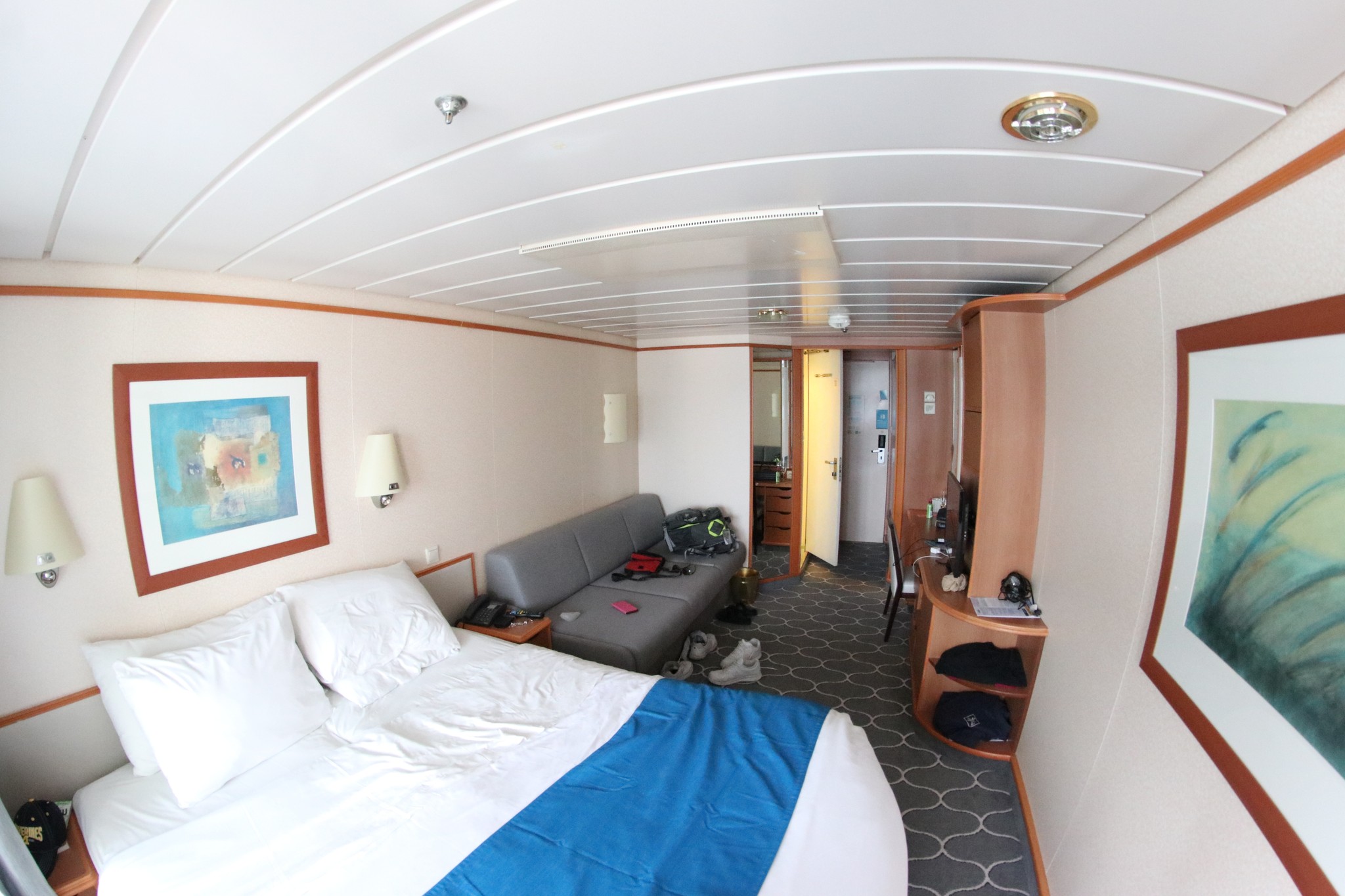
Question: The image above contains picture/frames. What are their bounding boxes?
[112,361,331,597]
[1138,293,1345,896]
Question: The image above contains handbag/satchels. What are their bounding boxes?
[624,548,666,575]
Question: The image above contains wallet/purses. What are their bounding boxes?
[612,600,637,614]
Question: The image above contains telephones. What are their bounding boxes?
[454,594,507,629]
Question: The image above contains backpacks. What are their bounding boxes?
[662,507,739,562]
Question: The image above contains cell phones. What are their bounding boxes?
[612,600,638,614]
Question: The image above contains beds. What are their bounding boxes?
[73,624,911,896]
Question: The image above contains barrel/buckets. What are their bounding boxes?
[732,567,759,605]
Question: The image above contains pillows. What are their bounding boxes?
[74,558,476,810]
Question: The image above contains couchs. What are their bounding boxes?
[485,492,747,678]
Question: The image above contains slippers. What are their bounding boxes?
[715,603,758,625]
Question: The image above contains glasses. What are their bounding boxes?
[668,564,696,577]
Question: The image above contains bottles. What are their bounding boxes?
[776,468,781,482]
[926,497,933,519]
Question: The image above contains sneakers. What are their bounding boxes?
[659,661,693,681]
[689,631,718,660]
[719,638,762,669]
[708,657,762,685]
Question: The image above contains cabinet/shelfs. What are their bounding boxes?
[945,293,1070,599]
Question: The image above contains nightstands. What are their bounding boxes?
[44,808,99,896]
[457,616,553,651]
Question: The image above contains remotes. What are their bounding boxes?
[504,609,545,619]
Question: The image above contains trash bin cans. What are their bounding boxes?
[731,567,759,605]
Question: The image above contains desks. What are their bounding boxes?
[753,478,792,546]
[905,508,1048,762]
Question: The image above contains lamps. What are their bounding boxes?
[3,473,88,588]
[351,432,407,509]
[828,316,851,329]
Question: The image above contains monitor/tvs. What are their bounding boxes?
[944,470,969,579]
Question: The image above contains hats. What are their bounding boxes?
[12,799,67,880]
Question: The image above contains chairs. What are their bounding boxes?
[881,509,920,643]
